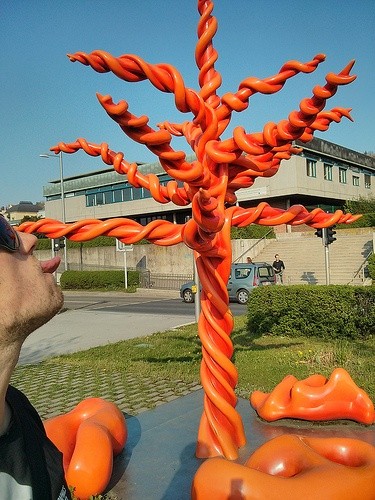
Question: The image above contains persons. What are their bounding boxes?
[273,254,285,285]
[0,213,71,500]
[247,257,252,263]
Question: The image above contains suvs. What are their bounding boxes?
[178,262,277,305]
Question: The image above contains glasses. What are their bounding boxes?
[0,213,21,253]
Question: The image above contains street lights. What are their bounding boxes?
[39,154,69,272]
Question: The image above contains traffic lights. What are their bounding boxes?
[59,237,65,248]
[327,225,337,244]
[314,228,321,237]
